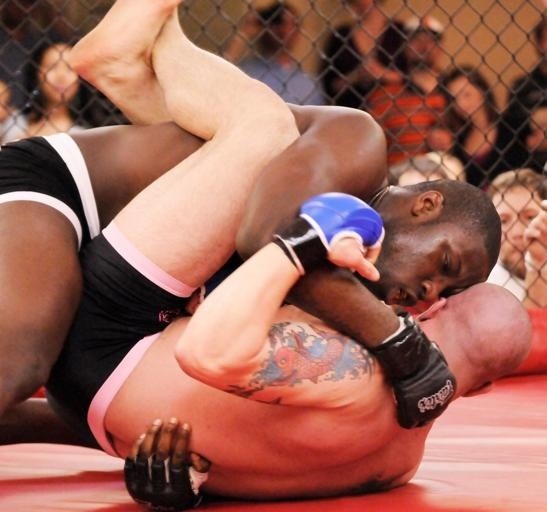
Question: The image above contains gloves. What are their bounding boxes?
[369,318,456,427]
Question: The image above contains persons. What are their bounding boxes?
[42,0,531,500]
[239,0,547,305]
[1,36,97,143]
[0,102,500,511]
